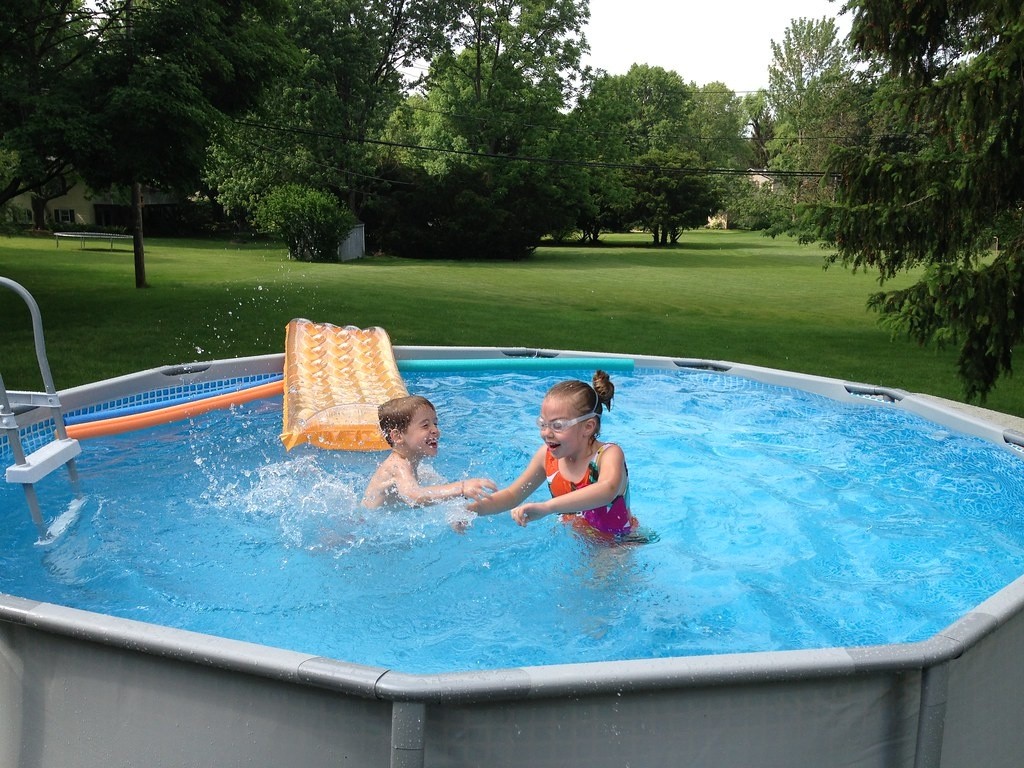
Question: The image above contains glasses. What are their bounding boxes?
[536,412,602,433]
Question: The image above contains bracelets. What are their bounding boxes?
[461,481,467,499]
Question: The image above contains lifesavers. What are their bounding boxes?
[55,373,285,440]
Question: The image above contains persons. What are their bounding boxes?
[361,397,497,506]
[467,370,630,530]
